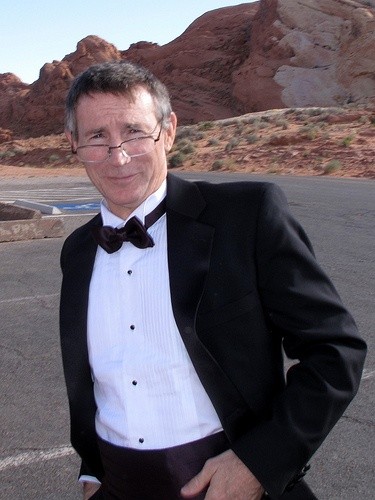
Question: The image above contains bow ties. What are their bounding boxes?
[95,194,168,255]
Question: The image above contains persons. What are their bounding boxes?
[57,60,369,500]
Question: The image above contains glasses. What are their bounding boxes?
[70,114,166,163]
